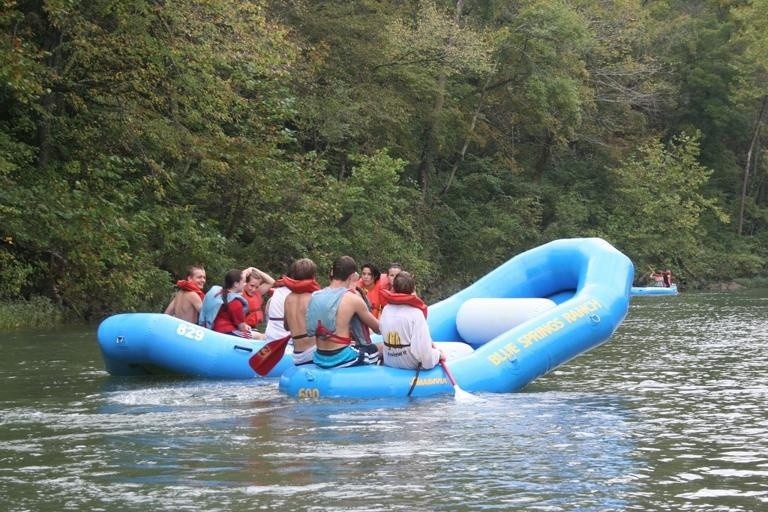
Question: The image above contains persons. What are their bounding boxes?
[164,256,443,370]
[649,269,673,288]
[227,340,230,342]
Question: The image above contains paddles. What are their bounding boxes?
[441,360,483,409]
[249,334,293,376]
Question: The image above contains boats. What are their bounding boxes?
[96,312,294,378]
[276,236,636,400]
[630,282,680,296]
